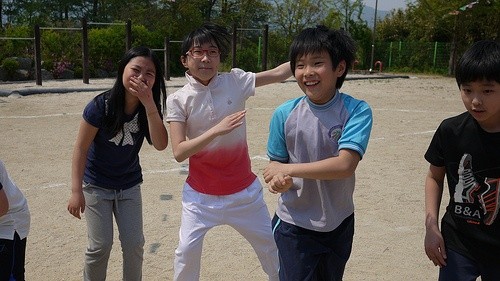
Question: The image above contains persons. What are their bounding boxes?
[0,159,30,281]
[424,33,500,281]
[261,25,374,281]
[67,46,169,281]
[166,26,296,281]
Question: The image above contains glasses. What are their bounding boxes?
[185,48,221,58]
